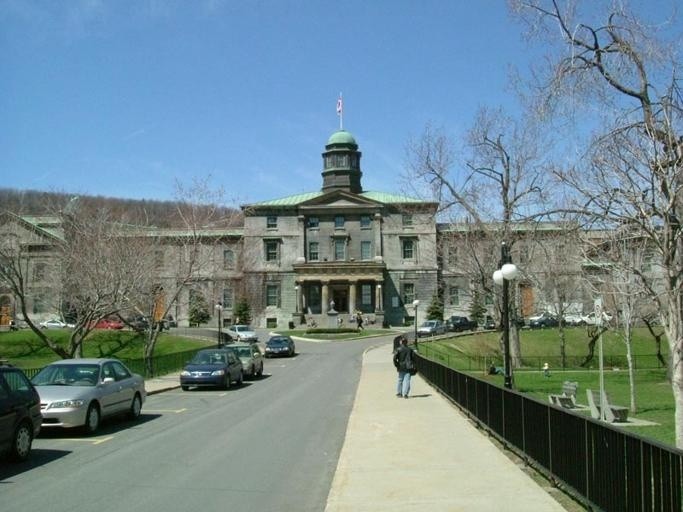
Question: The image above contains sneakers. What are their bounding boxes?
[396,393,408,398]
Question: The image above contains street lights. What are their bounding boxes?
[493,238,522,387]
[376,283,381,312]
[294,285,301,315]
[412,296,423,351]
[215,302,223,345]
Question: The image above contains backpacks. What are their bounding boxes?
[405,350,421,374]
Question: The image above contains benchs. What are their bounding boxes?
[586,387,629,422]
[547,380,579,408]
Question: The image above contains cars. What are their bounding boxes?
[419,312,622,335]
[179,325,301,392]
[37,316,162,330]
[17,358,148,434]
[0,360,42,463]
[10,320,29,331]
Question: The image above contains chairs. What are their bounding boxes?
[92,368,104,381]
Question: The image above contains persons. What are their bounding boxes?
[541,362,550,378]
[393,338,412,398]
[356,313,364,330]
[309,317,317,328]
[488,361,505,376]
[393,335,404,353]
[8,316,15,331]
